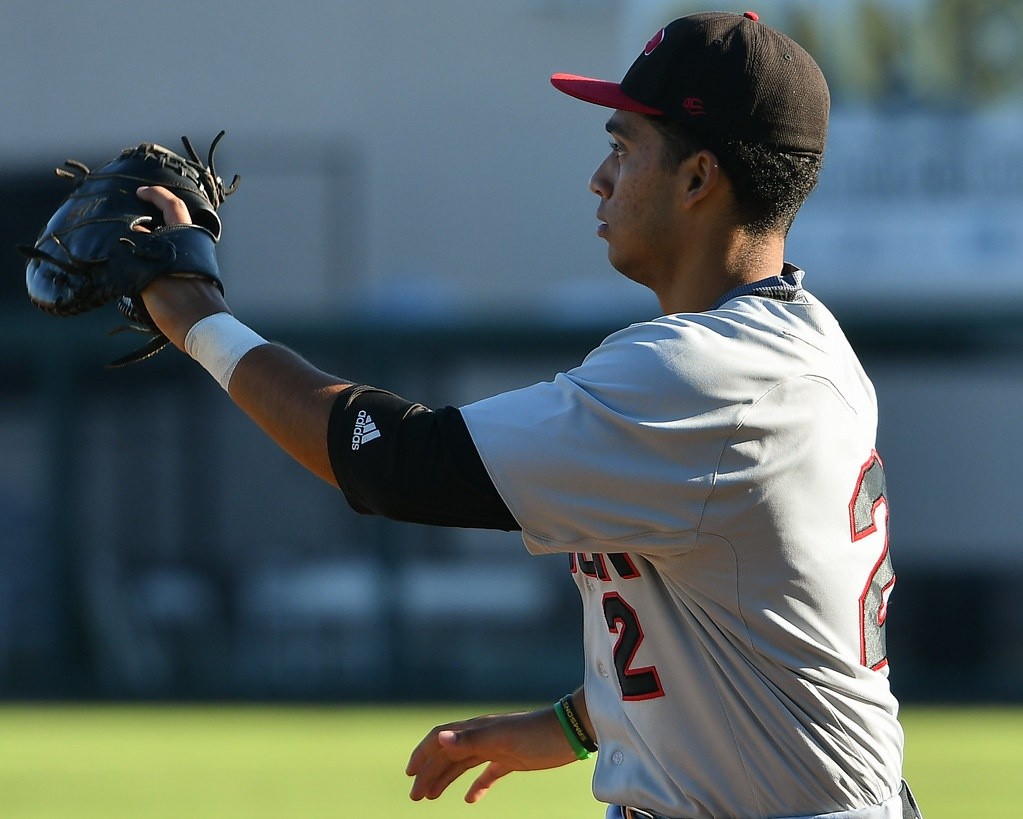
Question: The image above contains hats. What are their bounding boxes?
[549,11,829,156]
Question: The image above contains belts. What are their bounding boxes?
[620,781,917,819]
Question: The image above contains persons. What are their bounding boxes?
[27,12,922,819]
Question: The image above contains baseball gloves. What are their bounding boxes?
[12,123,244,374]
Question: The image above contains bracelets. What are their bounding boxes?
[553,693,599,760]
